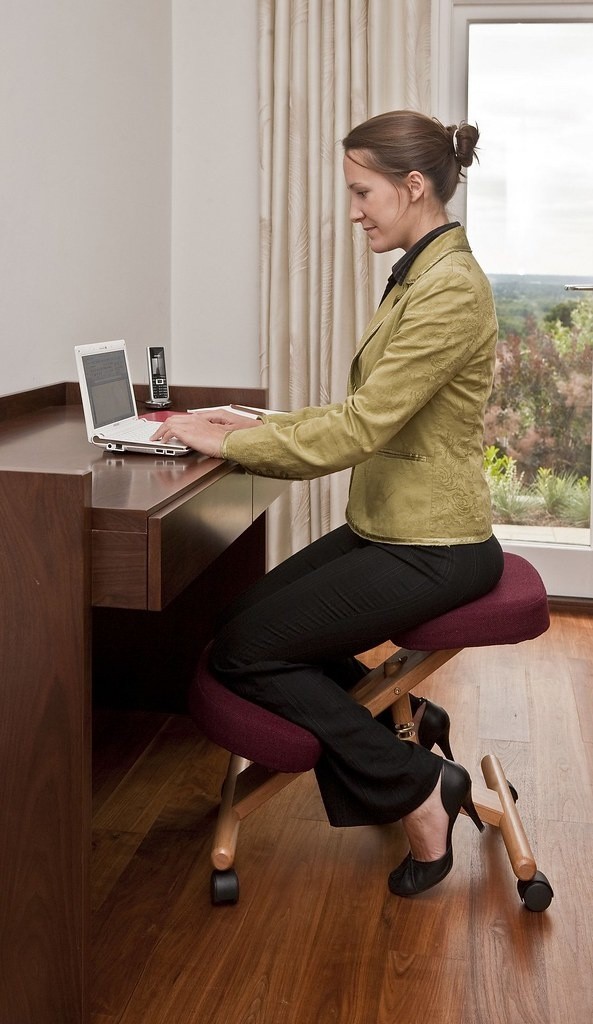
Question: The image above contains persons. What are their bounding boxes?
[153,109,503,897]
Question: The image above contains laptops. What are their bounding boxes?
[75,340,194,456]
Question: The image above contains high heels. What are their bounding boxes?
[417,700,454,762]
[388,759,485,896]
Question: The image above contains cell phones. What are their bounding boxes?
[147,346,169,403]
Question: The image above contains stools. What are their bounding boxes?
[188,551,554,913]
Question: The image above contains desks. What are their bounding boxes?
[0,382,293,1024]
